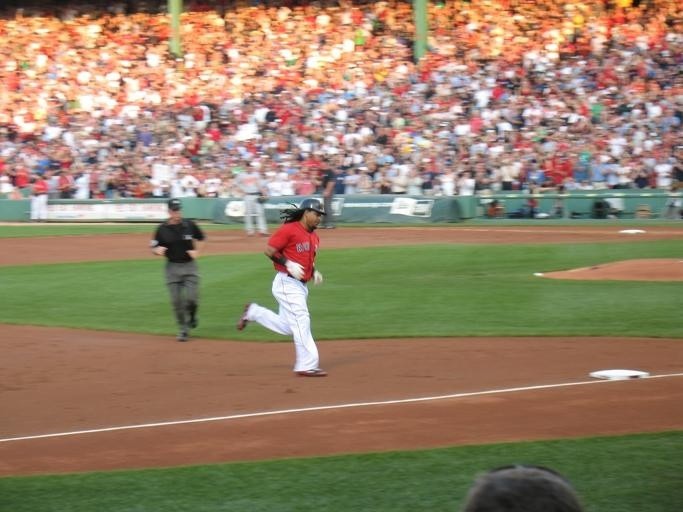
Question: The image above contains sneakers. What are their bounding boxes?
[246,230,272,237]
[315,225,335,229]
[237,302,253,331]
[175,313,197,342]
[296,367,328,377]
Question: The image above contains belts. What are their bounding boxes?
[286,273,306,284]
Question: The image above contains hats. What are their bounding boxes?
[167,199,181,210]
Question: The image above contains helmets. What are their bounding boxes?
[297,198,326,216]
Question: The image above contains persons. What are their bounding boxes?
[0,1,683,223]
[149,196,206,343]
[228,161,271,238]
[236,195,329,378]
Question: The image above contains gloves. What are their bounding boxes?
[311,269,322,286]
[283,260,304,282]
